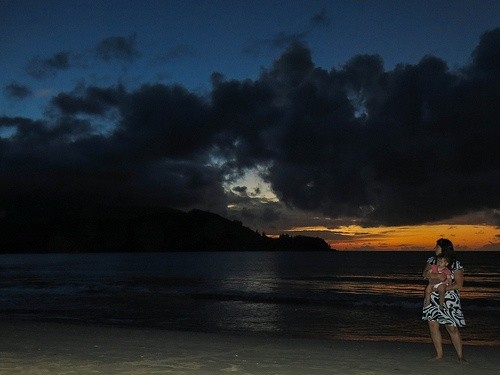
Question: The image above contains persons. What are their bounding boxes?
[422,238,467,362]
[424,254,453,310]
